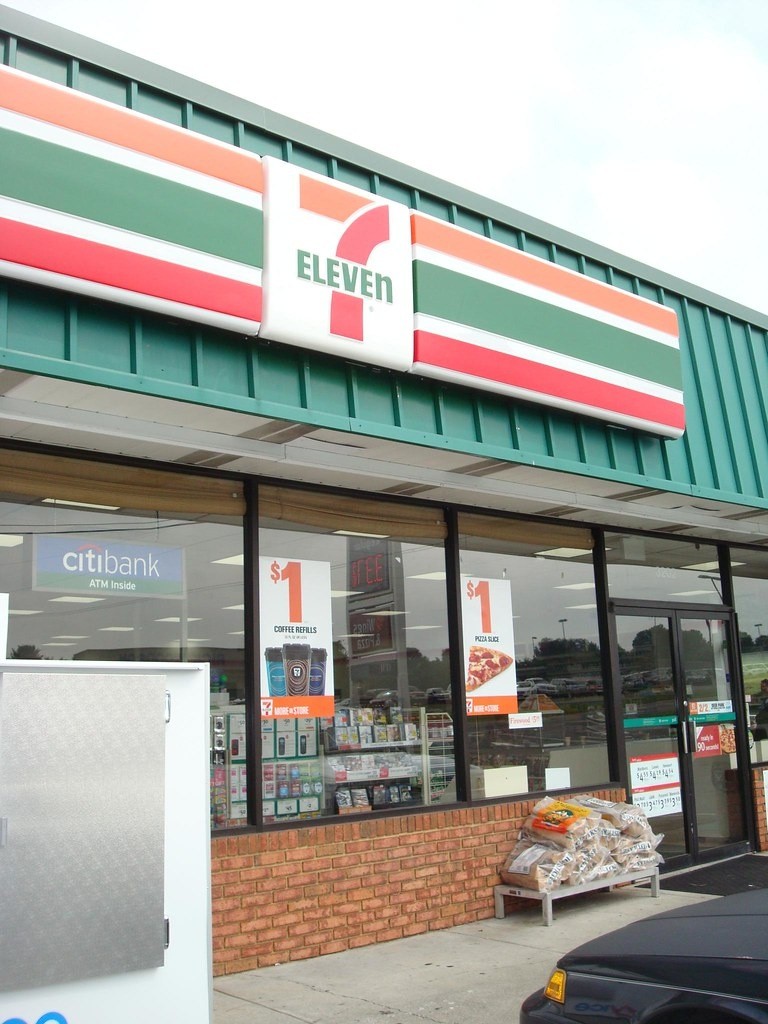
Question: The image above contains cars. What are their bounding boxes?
[334,683,397,709]
[584,668,730,696]
[516,681,533,700]
[425,688,450,701]
[520,885,768,1024]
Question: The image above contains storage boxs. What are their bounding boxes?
[319,705,418,750]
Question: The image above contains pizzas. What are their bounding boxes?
[721,728,737,753]
[466,645,513,692]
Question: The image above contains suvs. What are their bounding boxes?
[525,678,559,696]
[552,678,585,698]
[408,686,427,706]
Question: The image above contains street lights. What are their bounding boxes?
[533,636,538,664]
[558,619,568,656]
[754,623,762,636]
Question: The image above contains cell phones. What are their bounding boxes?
[278,737,285,756]
[231,739,239,755]
[300,736,307,754]
